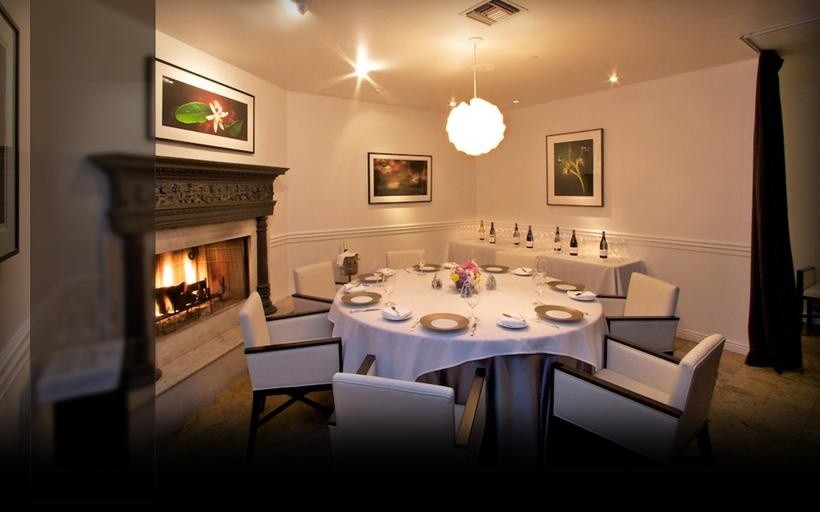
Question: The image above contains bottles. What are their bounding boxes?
[478,219,608,259]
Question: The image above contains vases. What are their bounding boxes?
[455,280,473,293]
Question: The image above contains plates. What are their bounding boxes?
[341,264,585,331]
[428,267,430,268]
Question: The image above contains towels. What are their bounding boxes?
[380,302,412,322]
[566,287,597,300]
[497,310,528,329]
[335,248,361,267]
[511,266,533,275]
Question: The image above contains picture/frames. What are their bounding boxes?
[147,56,255,153]
[368,153,433,203]
[547,127,604,207]
[0,5,20,263]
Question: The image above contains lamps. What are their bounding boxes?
[445,36,506,156]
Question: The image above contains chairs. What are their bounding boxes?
[237,292,343,446]
[797,267,820,339]
[594,272,680,357]
[328,353,486,475]
[291,261,342,311]
[386,248,426,270]
[548,332,726,465]
[494,250,532,270]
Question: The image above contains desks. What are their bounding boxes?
[448,233,647,298]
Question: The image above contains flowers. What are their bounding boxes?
[451,260,481,298]
